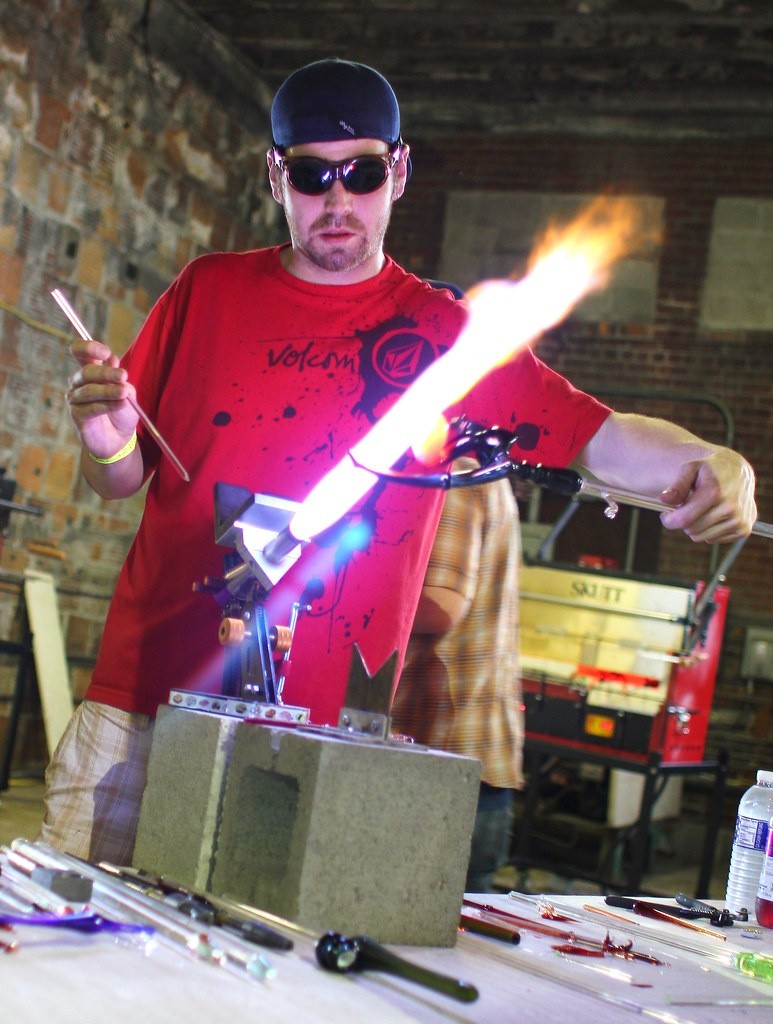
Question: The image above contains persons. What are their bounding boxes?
[31,60,759,869]
[390,281,529,897]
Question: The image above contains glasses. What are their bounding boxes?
[273,147,399,196]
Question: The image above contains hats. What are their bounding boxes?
[270,59,411,182]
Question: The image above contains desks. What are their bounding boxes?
[0,894,773,1024]
[491,735,730,896]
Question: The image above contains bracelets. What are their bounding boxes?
[87,428,138,464]
